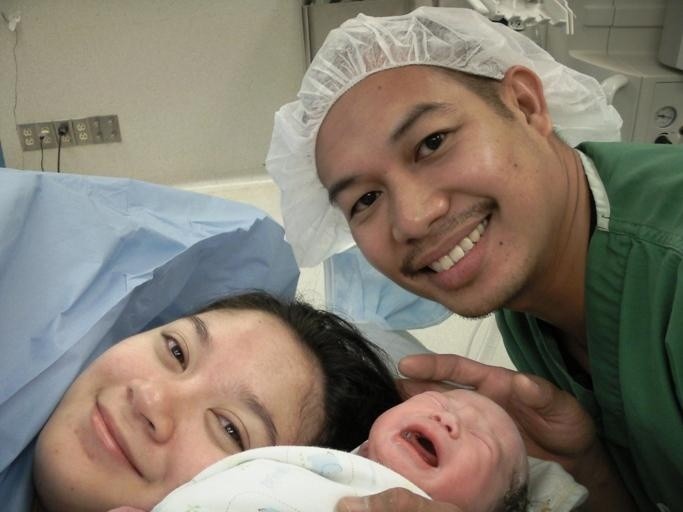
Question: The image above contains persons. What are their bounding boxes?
[30,287,403,512]
[264,2,683,511]
[357,383,532,511]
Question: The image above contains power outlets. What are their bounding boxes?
[16,114,122,153]
